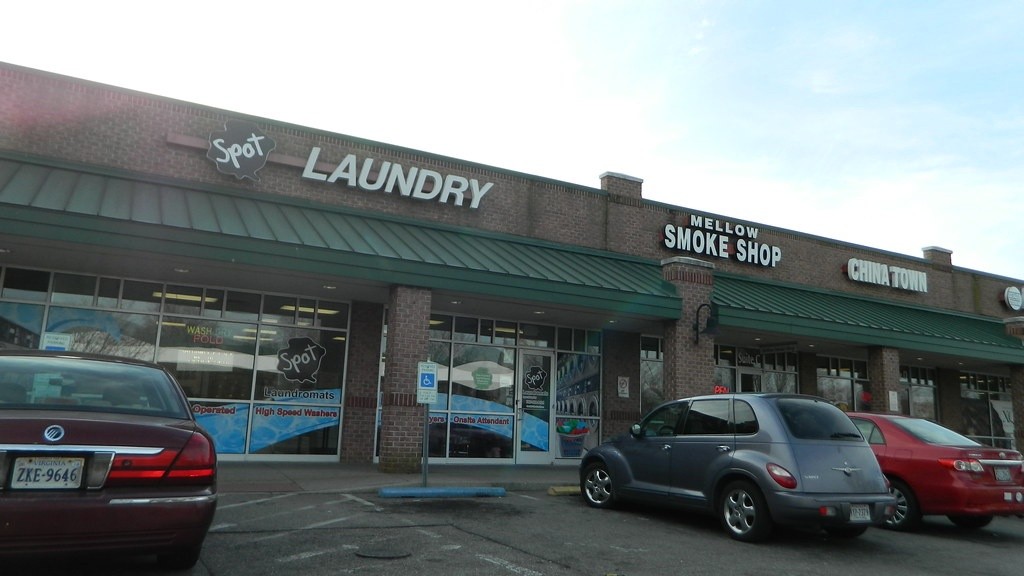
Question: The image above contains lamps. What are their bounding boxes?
[694,303,721,345]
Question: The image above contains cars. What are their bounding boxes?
[844,409,1024,535]
[0,344,221,571]
[576,391,900,543]
